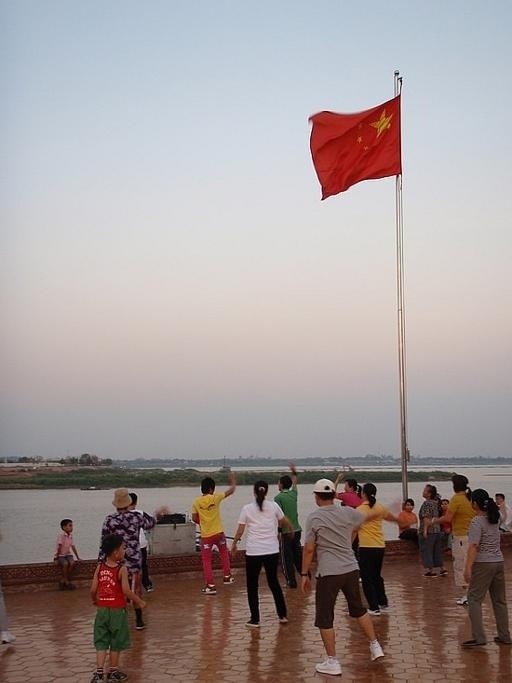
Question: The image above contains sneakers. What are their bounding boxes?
[367,603,388,616]
[107,671,127,683]
[316,659,342,676]
[136,623,144,630]
[245,620,260,628]
[422,569,448,578]
[456,598,468,605]
[200,585,217,595]
[90,673,104,683]
[280,617,289,623]
[494,636,510,643]
[463,640,485,646]
[1,635,16,644]
[59,582,74,591]
[370,643,384,662]
[223,575,235,585]
[146,585,154,592]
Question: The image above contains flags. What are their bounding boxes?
[308,95,401,200]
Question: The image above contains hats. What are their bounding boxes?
[313,479,335,493]
[113,489,133,508]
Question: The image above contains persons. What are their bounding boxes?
[54,518,80,588]
[334,473,365,582]
[272,460,311,589]
[301,479,385,676]
[0,583,15,643]
[230,477,294,627]
[89,491,157,683]
[398,475,511,605]
[192,473,236,595]
[461,488,512,646]
[355,483,398,616]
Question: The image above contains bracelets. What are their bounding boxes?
[278,527,282,533]
[302,572,308,577]
